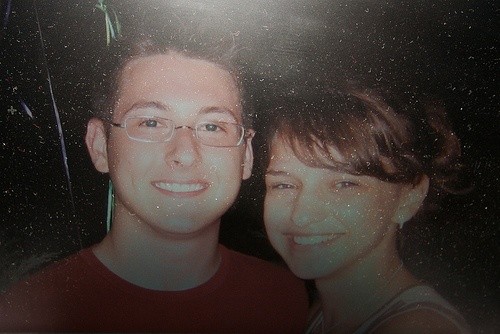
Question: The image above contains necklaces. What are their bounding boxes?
[317,260,405,330]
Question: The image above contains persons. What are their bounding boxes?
[1,2,318,333]
[250,38,481,334]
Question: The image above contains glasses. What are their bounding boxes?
[107,115,258,148]
